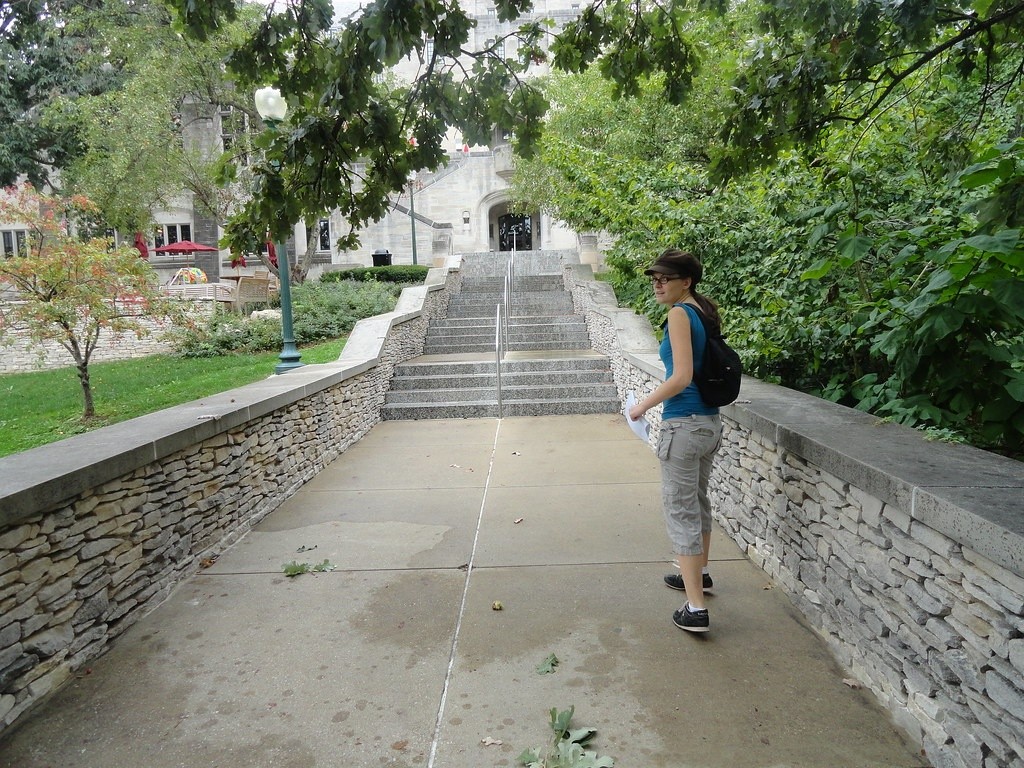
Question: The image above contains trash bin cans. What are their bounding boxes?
[371,249,392,266]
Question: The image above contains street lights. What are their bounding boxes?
[406,168,418,266]
[254,84,305,376]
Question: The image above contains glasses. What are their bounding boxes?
[649,277,681,284]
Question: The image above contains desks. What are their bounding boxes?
[217,275,254,290]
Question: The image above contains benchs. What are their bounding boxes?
[213,271,271,318]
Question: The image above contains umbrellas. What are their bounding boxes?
[232,256,247,276]
[132,228,151,257]
[266,229,278,273]
[149,238,219,282]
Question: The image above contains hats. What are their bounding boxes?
[644,249,703,284]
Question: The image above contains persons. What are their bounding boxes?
[629,248,725,632]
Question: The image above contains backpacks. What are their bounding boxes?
[660,303,743,407]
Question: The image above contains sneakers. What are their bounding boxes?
[664,573,713,592]
[673,602,710,632]
